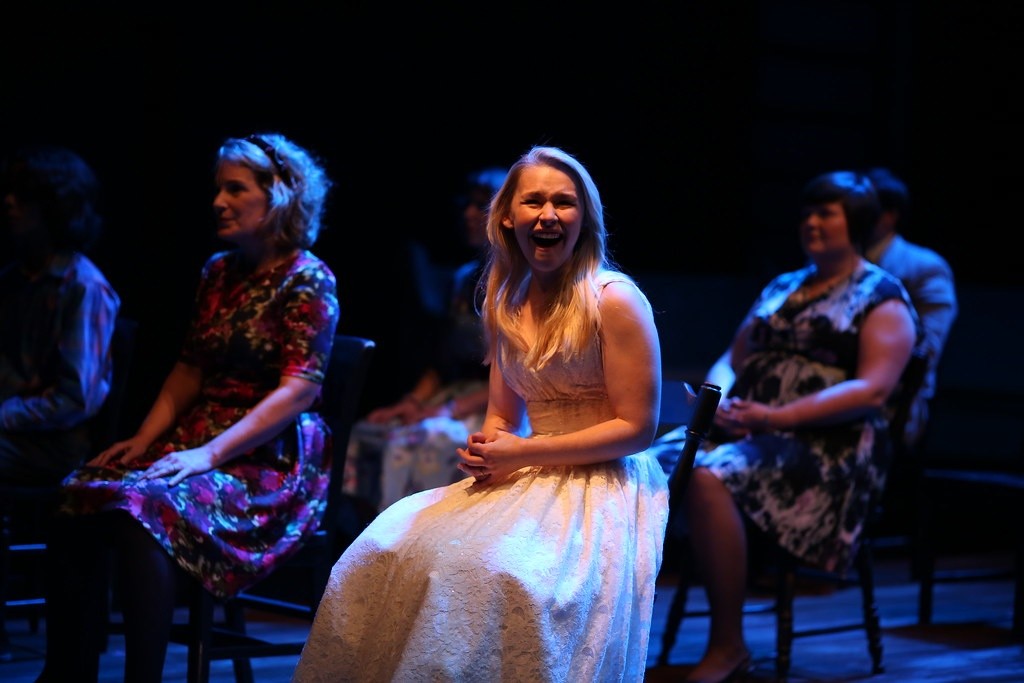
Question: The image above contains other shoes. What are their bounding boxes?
[682,653,749,683]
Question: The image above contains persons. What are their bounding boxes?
[291,146,670,683]
[0,128,535,683]
[644,166,957,683]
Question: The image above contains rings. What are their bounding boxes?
[480,468,484,479]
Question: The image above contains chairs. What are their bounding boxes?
[0,335,377,683]
[658,352,1024,683]
